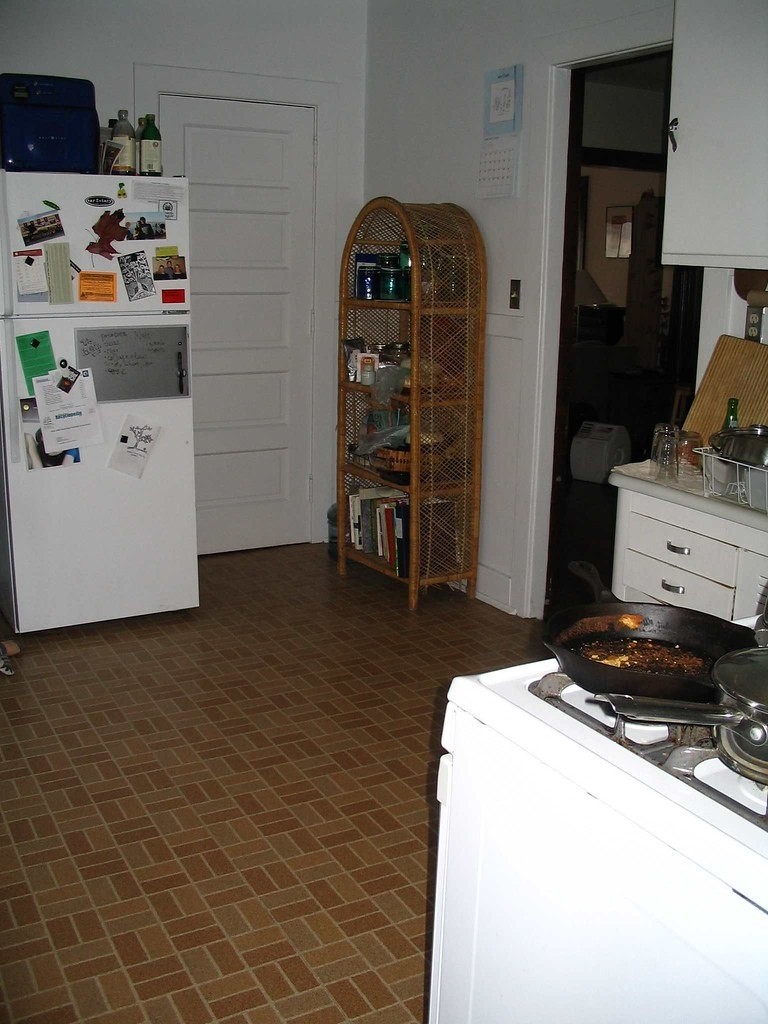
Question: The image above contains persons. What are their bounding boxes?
[124,217,154,239]
[22,217,54,241]
[24,428,79,470]
[154,261,185,280]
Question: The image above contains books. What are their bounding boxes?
[346,487,457,577]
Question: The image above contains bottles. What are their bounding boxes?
[327,502,338,558]
[341,241,466,393]
[720,398,739,429]
[102,109,163,176]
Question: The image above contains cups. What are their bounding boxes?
[649,423,704,485]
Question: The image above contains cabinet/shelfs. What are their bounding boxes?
[336,194,486,614]
[605,367,676,451]
[660,0,767,257]
[611,489,739,622]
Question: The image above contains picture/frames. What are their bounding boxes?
[605,205,633,258]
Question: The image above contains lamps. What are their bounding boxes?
[575,270,607,342]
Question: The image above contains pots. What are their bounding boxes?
[708,423,768,468]
[593,644,768,786]
[539,603,759,704]
